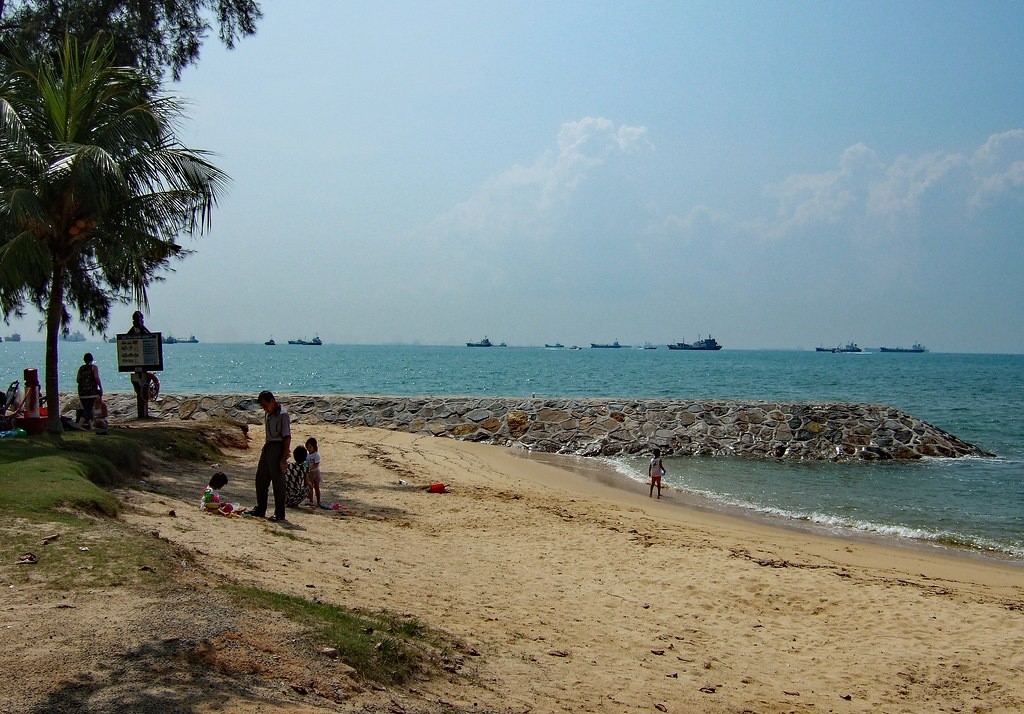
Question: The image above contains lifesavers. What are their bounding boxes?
[133,372,160,398]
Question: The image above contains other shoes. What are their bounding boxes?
[76,409,82,422]
[244,509,265,517]
[268,514,285,521]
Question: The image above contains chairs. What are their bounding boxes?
[0,387,31,431]
[1,379,20,414]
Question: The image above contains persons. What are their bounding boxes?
[243,390,293,522]
[199,471,240,512]
[304,438,326,508]
[283,446,312,508]
[648,448,666,499]
[75,353,104,431]
[90,389,110,430]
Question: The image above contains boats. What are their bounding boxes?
[466,336,493,348]
[265,334,322,346]
[544,338,657,352]
[816,342,862,354]
[66,331,87,342]
[880,344,925,354]
[668,334,723,351]
[161,333,199,345]
[4,334,21,342]
